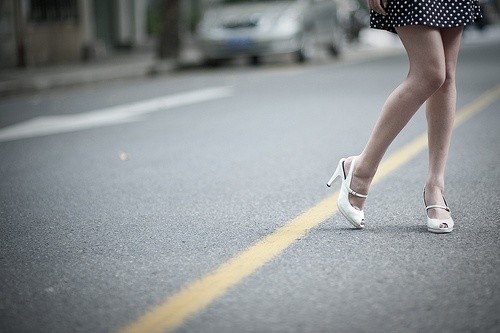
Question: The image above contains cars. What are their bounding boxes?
[193,1,343,66]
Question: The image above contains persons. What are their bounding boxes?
[325,0,484,234]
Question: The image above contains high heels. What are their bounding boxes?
[422,184,455,233]
[327,156,367,230]
